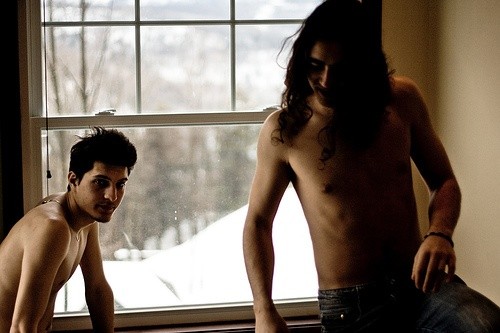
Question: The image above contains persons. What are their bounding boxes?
[0,125,138,332]
[244,0,500,332]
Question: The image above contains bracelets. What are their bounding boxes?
[425,231,455,248]
